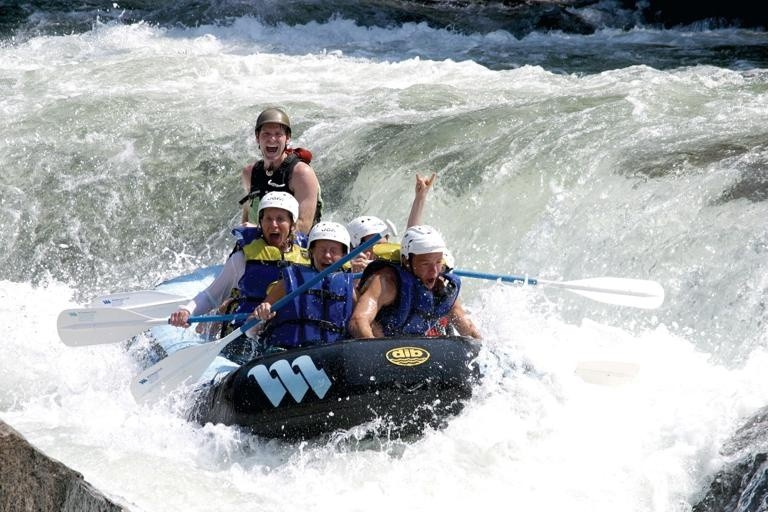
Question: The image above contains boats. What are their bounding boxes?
[125,261,529,474]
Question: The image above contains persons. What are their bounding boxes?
[249,220,358,358]
[241,107,324,238]
[169,191,311,367]
[348,224,482,342]
[346,170,438,304]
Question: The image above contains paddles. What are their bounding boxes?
[362,258,665,309]
[58,308,256,346]
[93,271,363,319]
[131,227,392,407]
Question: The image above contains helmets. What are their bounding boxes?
[400,226,454,274]
[257,191,299,224]
[347,216,390,248]
[307,222,350,254]
[255,109,291,132]
